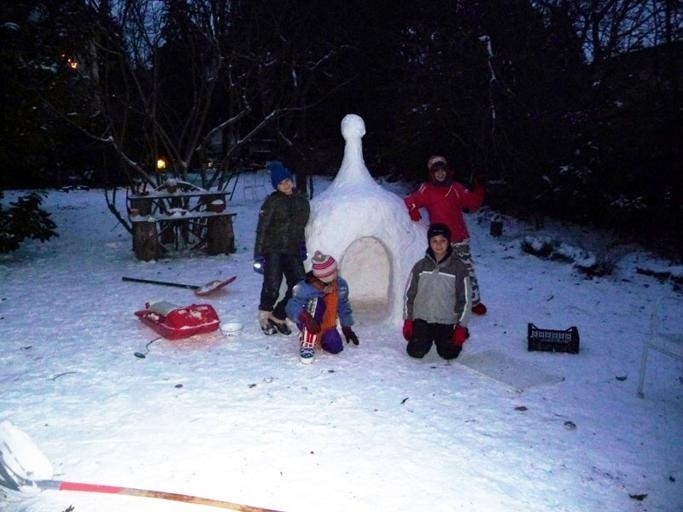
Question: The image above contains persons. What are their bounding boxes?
[402,223,472,360]
[284,250,359,364]
[404,155,487,316]
[253,160,308,336]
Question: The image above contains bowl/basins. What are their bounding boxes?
[221,322,243,339]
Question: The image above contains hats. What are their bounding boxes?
[266,162,294,190]
[311,251,337,279]
[427,154,450,185]
[427,225,451,243]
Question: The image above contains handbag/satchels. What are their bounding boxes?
[527,322,580,354]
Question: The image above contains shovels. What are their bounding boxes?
[122,275,236,296]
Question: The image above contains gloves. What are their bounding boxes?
[472,175,479,187]
[299,242,307,260]
[341,326,359,345]
[298,310,321,335]
[253,255,267,275]
[409,204,421,222]
[403,320,414,340]
[453,325,467,346]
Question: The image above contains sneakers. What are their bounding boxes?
[269,317,292,338]
[472,303,486,315]
[260,323,277,335]
[299,345,315,363]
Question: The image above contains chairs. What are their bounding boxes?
[638,296,683,401]
